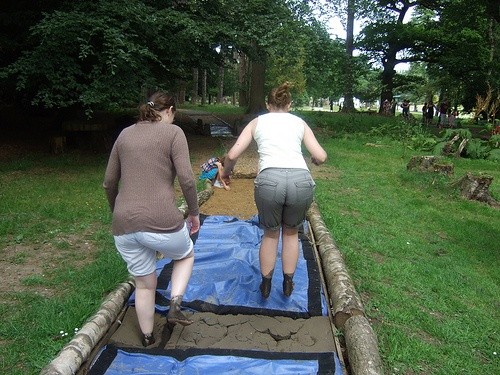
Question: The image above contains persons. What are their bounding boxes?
[103,92,201,347]
[200,153,231,191]
[219,81,327,298]
[400,99,410,120]
[439,100,454,128]
[422,100,434,125]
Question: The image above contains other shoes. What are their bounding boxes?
[214,179,224,188]
